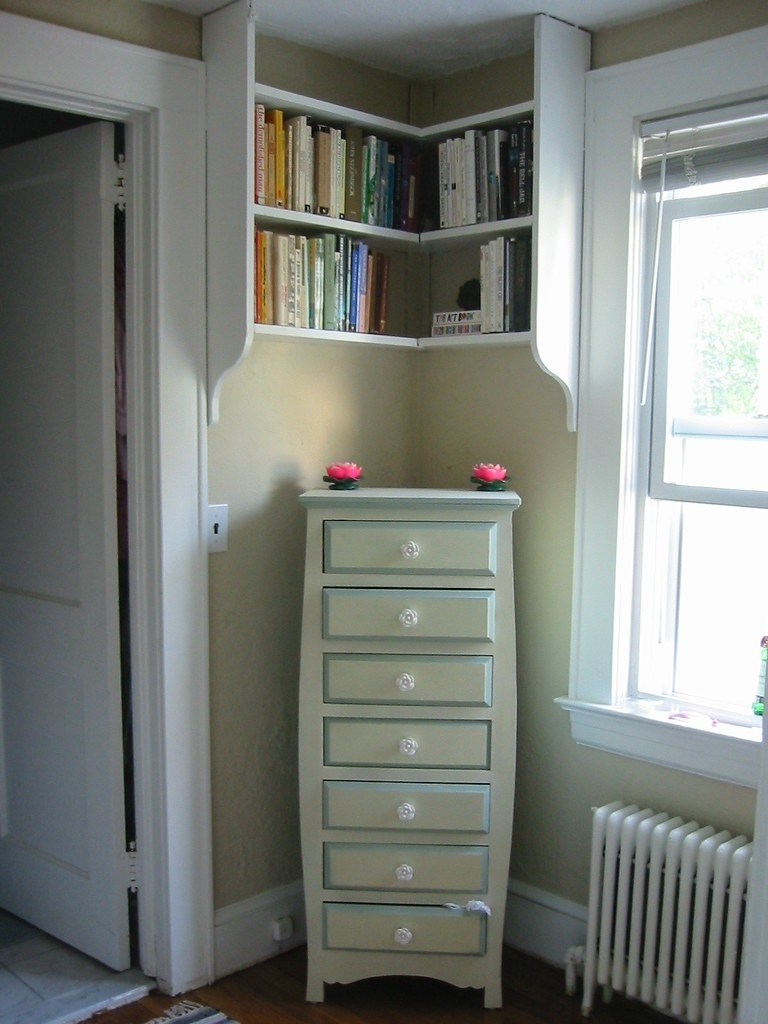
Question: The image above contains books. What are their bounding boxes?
[251,229,393,335]
[254,103,534,232]
[477,236,530,335]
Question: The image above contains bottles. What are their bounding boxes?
[752,633,768,715]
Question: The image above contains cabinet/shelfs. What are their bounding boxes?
[294,487,522,1009]
[202,0,590,437]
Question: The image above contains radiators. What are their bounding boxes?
[562,799,753,1024]
